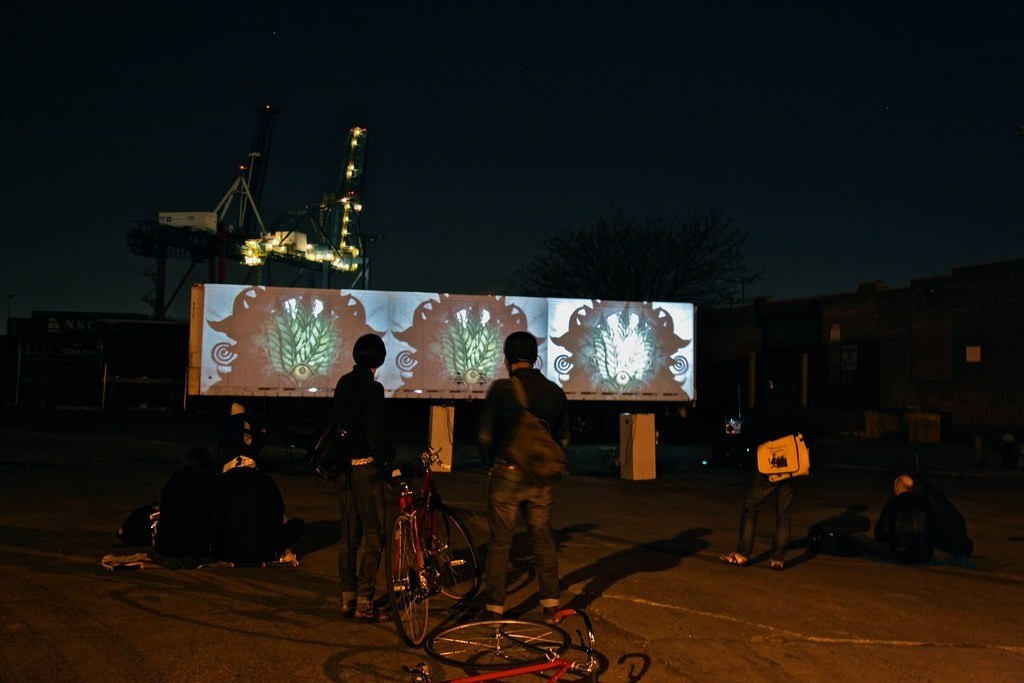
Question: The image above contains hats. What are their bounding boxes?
[353,334,386,368]
[504,332,538,363]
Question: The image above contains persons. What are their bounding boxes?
[719,365,807,571]
[115,436,285,567]
[327,334,397,623]
[475,332,569,624]
[861,474,973,563]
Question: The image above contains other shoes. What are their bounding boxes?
[355,604,390,622]
[482,609,503,628]
[341,600,356,617]
[543,607,558,625]
[719,553,747,566]
[771,560,783,569]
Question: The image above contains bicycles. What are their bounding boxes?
[370,448,482,649]
[401,609,602,683]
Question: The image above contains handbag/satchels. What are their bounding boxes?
[806,527,858,557]
[757,433,810,485]
[511,411,566,478]
[309,421,350,476]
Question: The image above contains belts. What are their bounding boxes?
[350,457,374,465]
